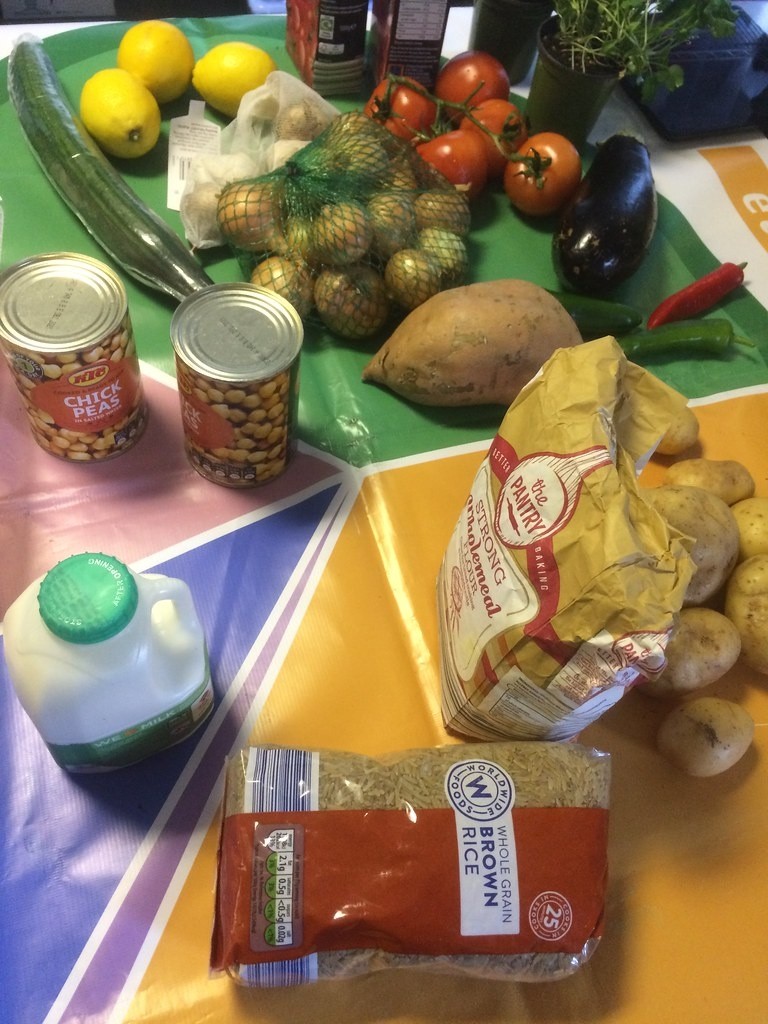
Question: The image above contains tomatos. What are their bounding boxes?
[365,51,583,217]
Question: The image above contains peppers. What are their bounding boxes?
[543,263,754,358]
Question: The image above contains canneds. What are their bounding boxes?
[1,251,304,488]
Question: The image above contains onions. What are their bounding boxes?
[218,108,472,340]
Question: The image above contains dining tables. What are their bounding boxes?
[0,4,768,1024]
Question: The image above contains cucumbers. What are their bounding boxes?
[8,33,218,302]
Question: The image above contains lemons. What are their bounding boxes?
[79,20,280,159]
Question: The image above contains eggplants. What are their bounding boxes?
[551,130,655,295]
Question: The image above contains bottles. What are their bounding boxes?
[0,549,218,777]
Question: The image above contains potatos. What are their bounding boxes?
[361,279,767,778]
